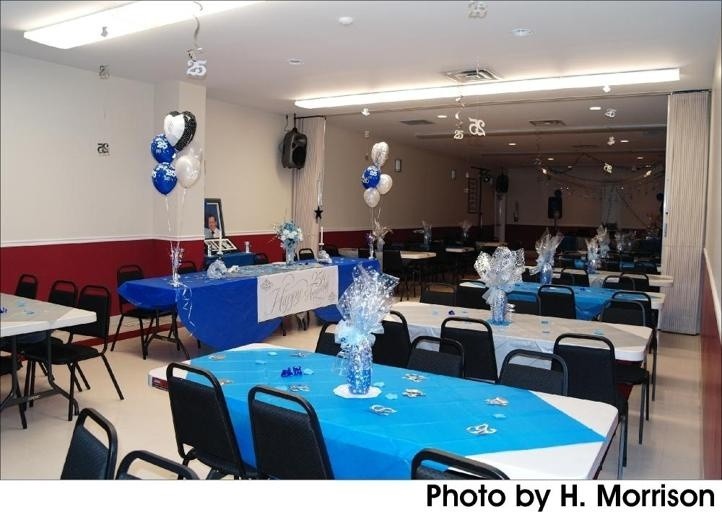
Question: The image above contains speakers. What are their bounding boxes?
[282,128,307,169]
[496,175,509,193]
[548,197,563,219]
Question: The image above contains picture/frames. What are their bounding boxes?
[204,195,226,241]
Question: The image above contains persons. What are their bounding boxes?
[203,213,221,240]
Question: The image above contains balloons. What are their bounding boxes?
[151,134,176,163]
[176,156,200,191]
[375,173,393,194]
[363,187,381,207]
[371,142,389,167]
[177,139,203,162]
[163,112,196,148]
[361,166,378,189]
[151,161,176,195]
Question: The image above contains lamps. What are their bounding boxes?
[21,2,262,51]
[412,123,668,141]
[291,65,678,111]
[474,146,666,158]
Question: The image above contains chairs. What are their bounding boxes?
[556,249,659,290]
[506,284,578,319]
[60,408,198,480]
[111,263,181,359]
[416,278,492,308]
[178,259,213,274]
[371,310,568,397]
[253,251,271,264]
[165,363,335,479]
[553,291,657,467]
[0,274,124,401]
[358,234,480,301]
[411,448,508,478]
[294,245,341,259]
